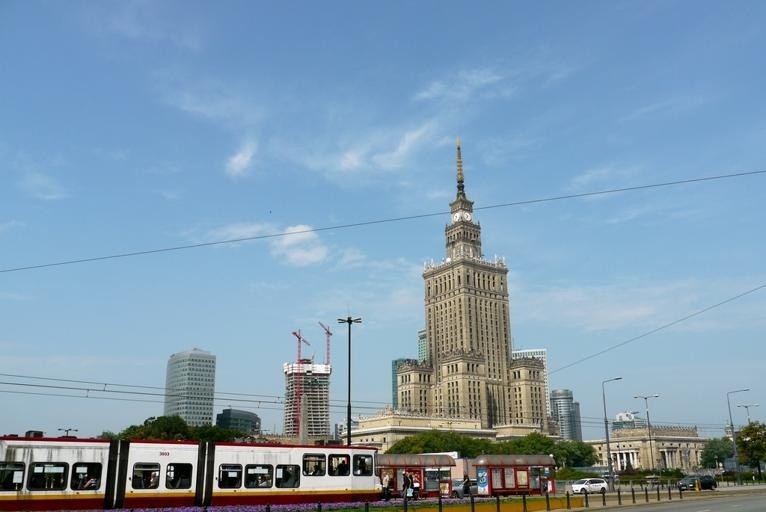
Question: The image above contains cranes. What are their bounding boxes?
[293,322,332,437]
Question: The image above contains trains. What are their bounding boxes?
[0,434,383,512]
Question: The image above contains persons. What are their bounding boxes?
[353,464,363,475]
[334,460,349,476]
[461,471,473,497]
[83,472,98,489]
[312,464,322,476]
[257,476,267,487]
[381,471,423,501]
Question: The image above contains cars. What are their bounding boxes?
[440,479,478,498]
[572,475,619,494]
[677,474,717,491]
[646,475,660,484]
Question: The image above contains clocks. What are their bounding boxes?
[453,213,460,222]
[464,212,472,221]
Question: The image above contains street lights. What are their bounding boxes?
[601,377,622,492]
[58,429,78,437]
[726,388,760,486]
[337,317,362,445]
[634,395,659,472]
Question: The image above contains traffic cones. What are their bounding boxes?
[695,479,701,492]
